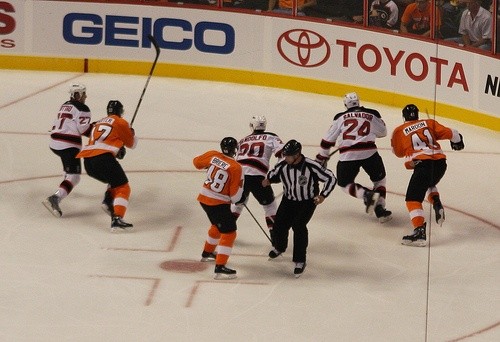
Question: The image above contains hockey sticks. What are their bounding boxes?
[130,34,161,128]
[242,203,283,261]
[424,108,431,119]
[274,148,340,199]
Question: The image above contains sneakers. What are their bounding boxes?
[401,221,426,247]
[374,204,392,223]
[102,191,113,216]
[41,194,63,217]
[294,261,308,278]
[433,200,445,227]
[267,248,287,261]
[362,187,380,216]
[200,251,217,262]
[213,265,237,280]
[110,215,133,232]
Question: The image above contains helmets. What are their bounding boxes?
[69,81,87,102]
[344,92,359,110]
[220,136,239,156]
[248,114,267,130]
[402,104,419,121]
[107,100,124,117]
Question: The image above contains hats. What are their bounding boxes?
[275,139,301,157]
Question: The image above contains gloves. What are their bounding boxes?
[315,154,330,168]
[451,133,464,151]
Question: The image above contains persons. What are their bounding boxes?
[230,115,284,257]
[193,137,244,279]
[42,80,105,218]
[262,139,337,278]
[315,92,392,224]
[391,104,465,247]
[75,101,137,232]
[180,0,500,53]
